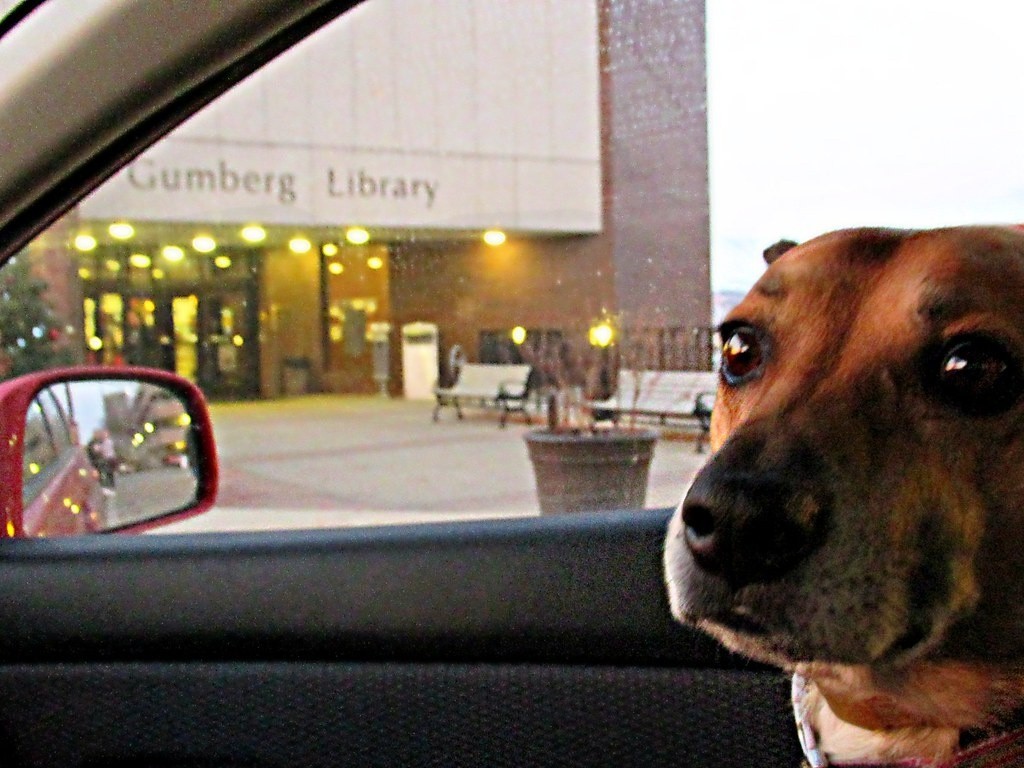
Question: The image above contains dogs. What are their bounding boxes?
[660,223,1022,768]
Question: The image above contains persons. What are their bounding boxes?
[432,333,622,441]
[86,427,118,497]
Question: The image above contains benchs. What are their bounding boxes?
[590,368,719,454]
[433,360,533,428]
